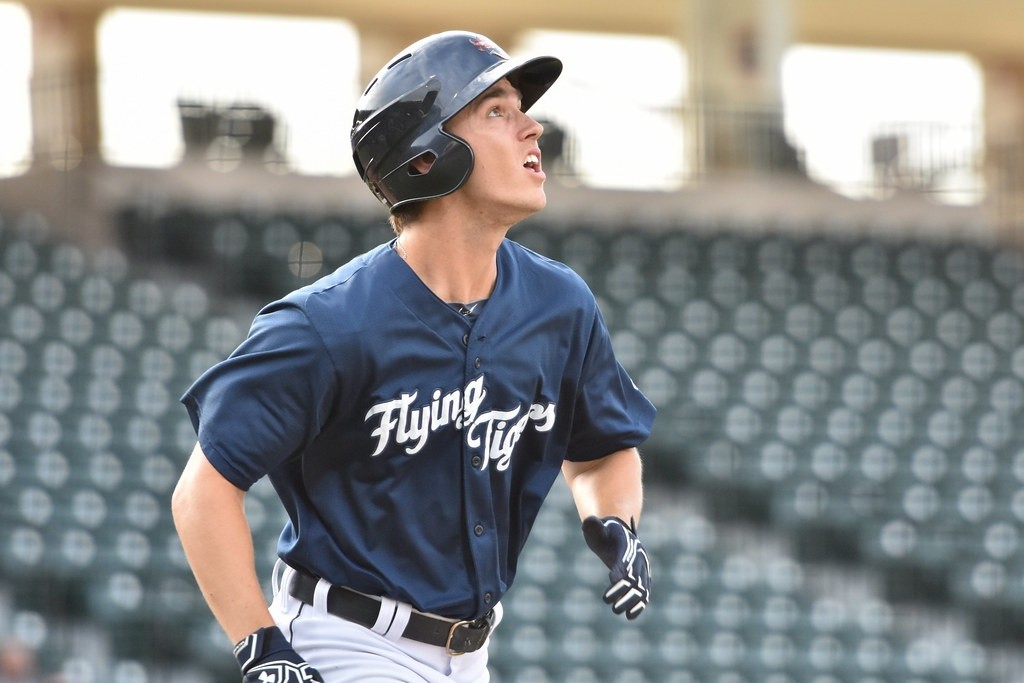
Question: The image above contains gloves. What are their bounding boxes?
[582,515,653,620]
[231,625,326,682]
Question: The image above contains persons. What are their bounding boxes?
[171,31,659,683]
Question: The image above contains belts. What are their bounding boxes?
[288,570,496,657]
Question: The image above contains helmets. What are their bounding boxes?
[349,30,564,213]
